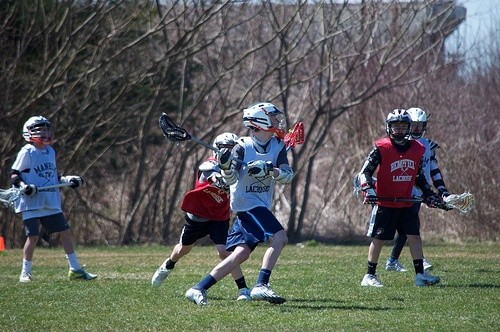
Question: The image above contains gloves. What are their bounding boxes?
[438,188,454,211]
[361,182,378,204]
[243,160,274,179]
[217,148,232,170]
[421,190,447,208]
[60,175,84,189]
[11,177,38,196]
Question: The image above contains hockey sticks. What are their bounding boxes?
[284,121,306,153]
[365,192,476,213]
[0,182,73,204]
[158,111,249,167]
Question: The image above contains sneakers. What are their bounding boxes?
[360,274,383,287]
[19,263,33,283]
[185,282,207,307]
[152,258,175,288]
[250,283,287,304]
[416,272,440,286]
[422,259,433,271]
[237,287,252,301]
[69,264,98,280]
[386,259,408,272]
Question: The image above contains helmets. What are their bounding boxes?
[213,132,240,159]
[22,115,53,144]
[385,108,413,146]
[406,106,428,140]
[243,101,284,131]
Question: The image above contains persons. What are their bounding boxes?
[151,132,252,300]
[185,102,294,306]
[358,108,443,288]
[387,108,452,273]
[10,115,98,283]
